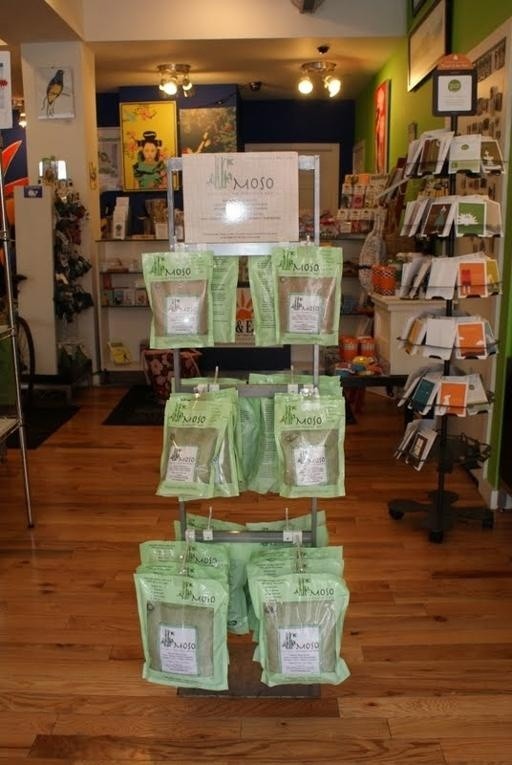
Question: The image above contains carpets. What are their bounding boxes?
[102,384,359,429]
[0,388,81,451]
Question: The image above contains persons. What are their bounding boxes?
[132,132,168,191]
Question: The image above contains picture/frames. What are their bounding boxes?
[411,0,425,18]
[119,100,180,192]
[406,0,450,93]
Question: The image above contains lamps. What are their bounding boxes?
[297,61,341,97]
[157,64,196,99]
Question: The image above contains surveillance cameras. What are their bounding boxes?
[317,45,330,53]
[248,80,261,92]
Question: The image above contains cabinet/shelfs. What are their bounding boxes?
[93,232,374,385]
[13,183,93,366]
[325,290,450,426]
